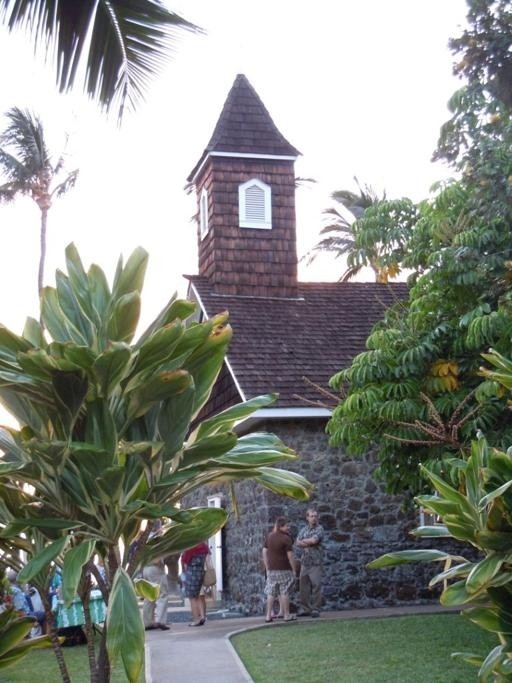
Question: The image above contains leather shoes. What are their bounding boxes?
[312,612,319,617]
[145,624,158,629]
[296,611,307,616]
[153,622,169,629]
[189,619,204,626]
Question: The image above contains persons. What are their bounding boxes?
[4,555,119,640]
[262,515,299,622]
[263,521,294,618]
[140,517,172,630]
[181,538,211,626]
[296,506,327,617]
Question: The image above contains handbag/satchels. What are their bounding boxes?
[202,568,216,586]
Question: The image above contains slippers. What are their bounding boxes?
[284,614,297,620]
[273,614,284,618]
[265,617,272,621]
